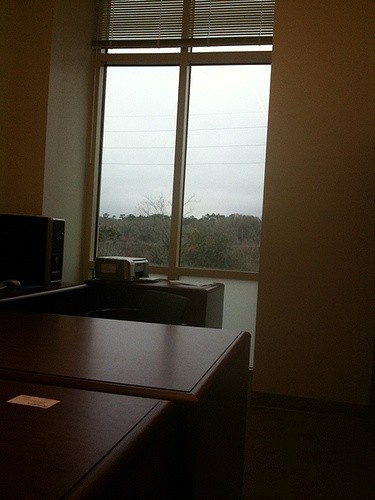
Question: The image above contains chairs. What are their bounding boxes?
[80,289,189,325]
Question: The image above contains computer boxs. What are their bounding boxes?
[0,212,65,282]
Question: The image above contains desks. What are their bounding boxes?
[0,306,252,500]
[0,280,89,319]
[87,278,226,329]
[0,376,185,500]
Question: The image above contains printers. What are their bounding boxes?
[96,256,149,282]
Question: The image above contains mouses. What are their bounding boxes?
[2,279,21,287]
